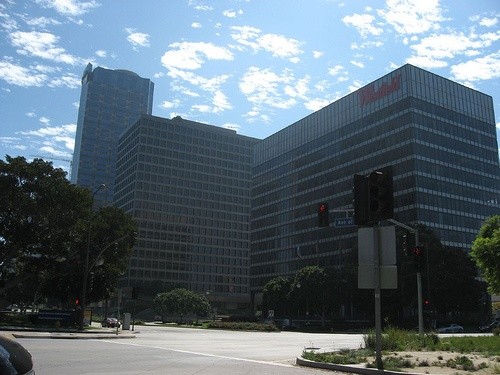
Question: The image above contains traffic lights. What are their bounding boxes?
[402,231,413,258]
[76,299,79,305]
[318,202,330,227]
[371,167,394,220]
[351,174,371,224]
[424,298,431,308]
[415,245,427,273]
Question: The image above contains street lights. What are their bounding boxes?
[205,290,215,320]
[297,281,309,320]
[80,183,106,331]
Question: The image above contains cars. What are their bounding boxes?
[102,318,121,328]
[436,324,465,333]
